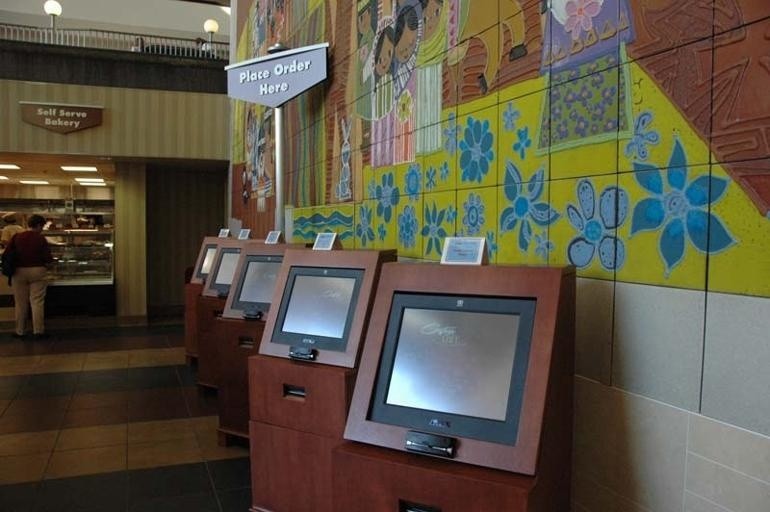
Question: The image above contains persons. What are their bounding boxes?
[2,216,56,339]
[1,214,27,249]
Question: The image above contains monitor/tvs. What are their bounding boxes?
[209,248,241,289]
[368,293,537,446]
[196,244,217,278]
[231,255,284,311]
[271,267,365,352]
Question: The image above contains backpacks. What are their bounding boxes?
[0,234,16,287]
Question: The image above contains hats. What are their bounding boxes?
[1,211,17,223]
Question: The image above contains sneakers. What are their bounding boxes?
[9,331,42,340]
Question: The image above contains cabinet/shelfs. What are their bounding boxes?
[29,230,114,316]
